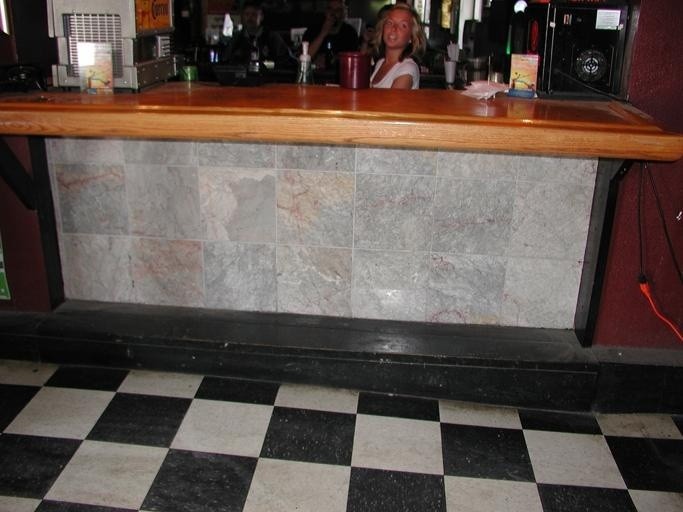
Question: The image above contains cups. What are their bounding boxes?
[443,61,457,84]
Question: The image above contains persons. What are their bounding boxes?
[366,4,423,89]
[300,2,359,84]
[360,0,420,60]
[218,4,299,83]
